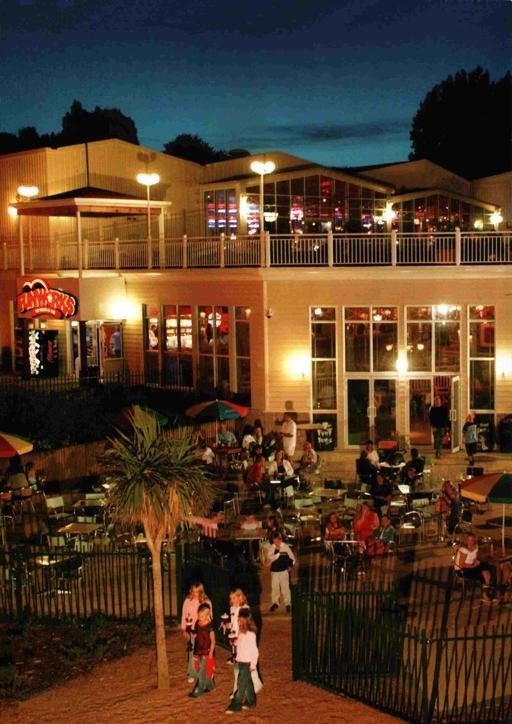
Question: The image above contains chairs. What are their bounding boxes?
[1,473,119,594]
[356,436,434,478]
[188,432,322,545]
[323,478,511,602]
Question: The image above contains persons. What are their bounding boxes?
[225,608,260,713]
[109,316,221,358]
[221,588,263,695]
[184,604,216,697]
[263,531,296,613]
[181,582,213,682]
[0,395,512,604]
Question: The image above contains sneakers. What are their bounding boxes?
[189,693,199,698]
[204,689,215,693]
[270,604,278,611]
[286,605,291,613]
[188,677,193,683]
